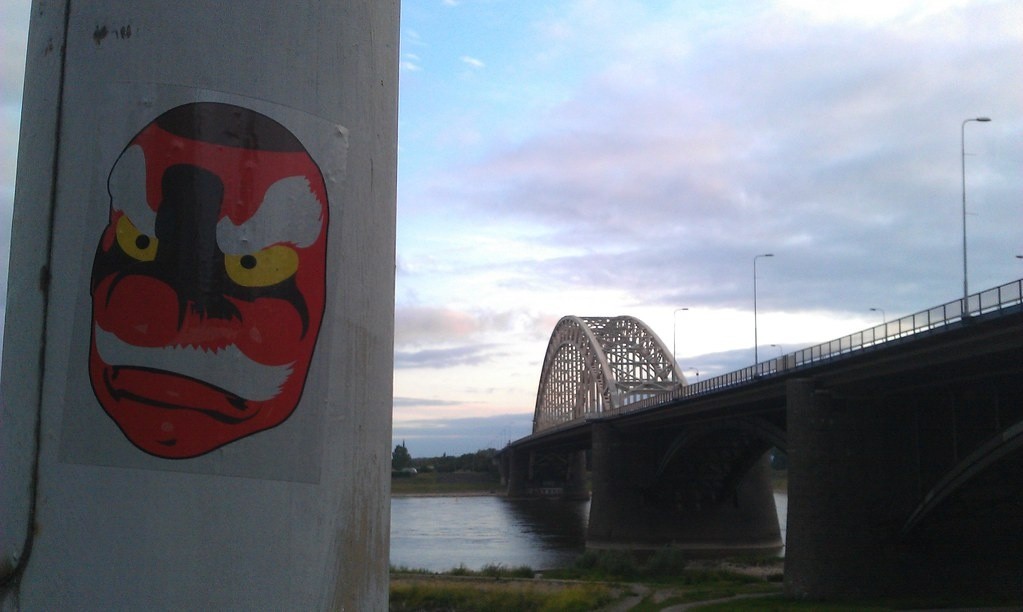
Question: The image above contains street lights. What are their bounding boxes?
[870,307,886,325]
[753,252,774,379]
[961,117,992,321]
[672,307,689,400]
[770,344,783,370]
[687,367,700,393]
